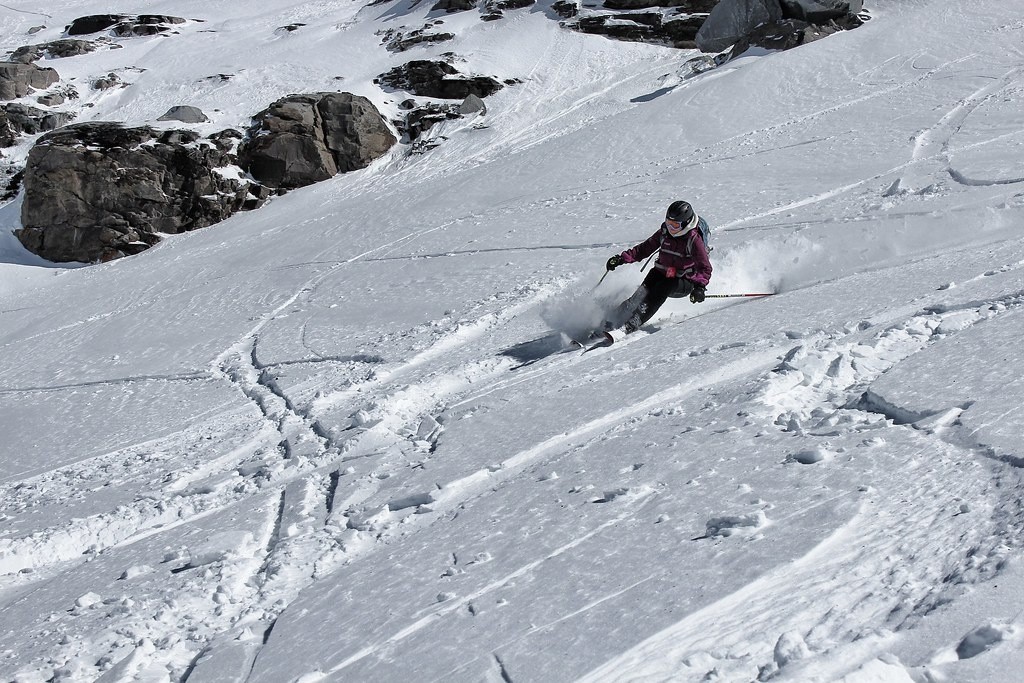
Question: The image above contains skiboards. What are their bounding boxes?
[570,298,634,349]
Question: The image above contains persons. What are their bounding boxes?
[589,201,712,335]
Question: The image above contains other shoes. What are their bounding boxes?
[586,319,638,344]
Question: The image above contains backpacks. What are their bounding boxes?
[697,217,709,245]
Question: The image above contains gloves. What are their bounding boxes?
[691,286,705,303]
[607,255,622,270]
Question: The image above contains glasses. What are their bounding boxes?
[664,218,686,230]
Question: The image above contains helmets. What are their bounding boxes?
[667,200,692,220]
